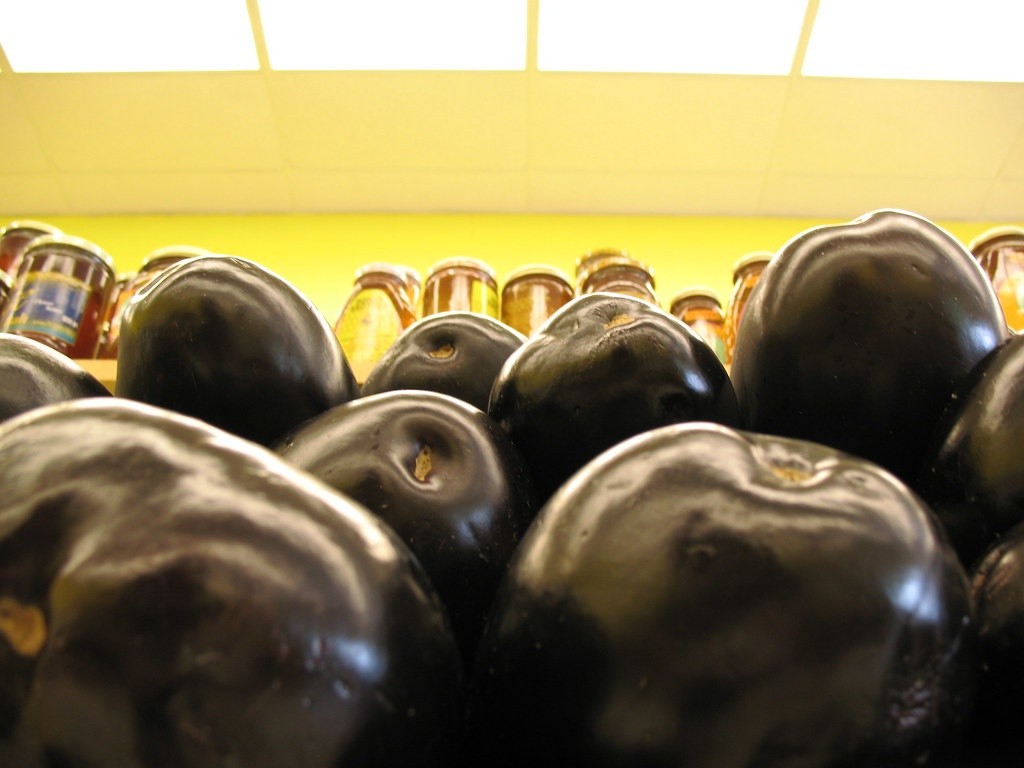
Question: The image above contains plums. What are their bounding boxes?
[0,208,1024,768]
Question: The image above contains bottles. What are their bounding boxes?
[332,263,417,386]
